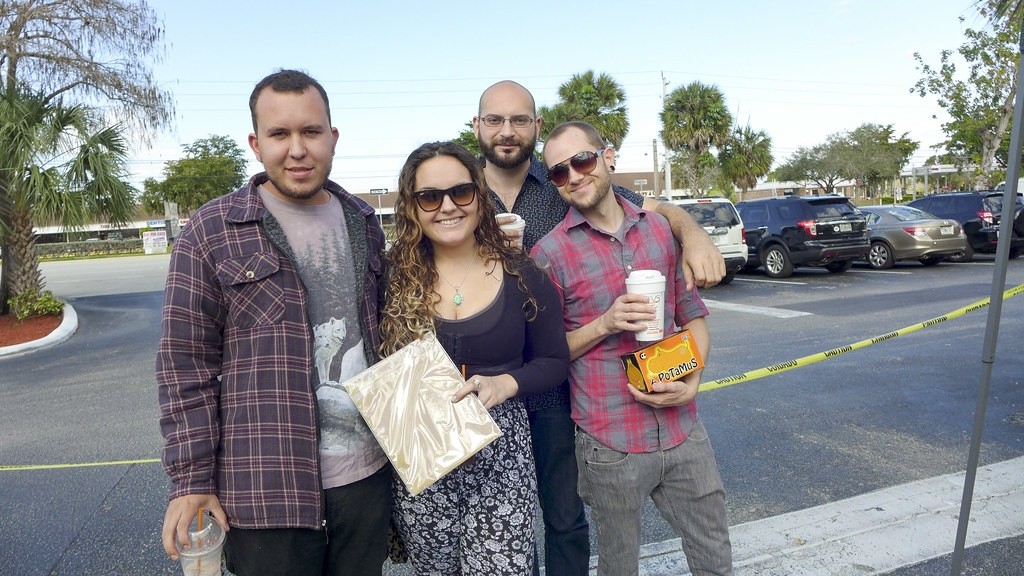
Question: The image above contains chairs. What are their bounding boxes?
[751,211,762,222]
[694,208,716,223]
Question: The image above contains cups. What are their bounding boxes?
[495,213,525,252]
[174,515,227,576]
[625,270,666,341]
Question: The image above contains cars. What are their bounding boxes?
[856,205,969,270]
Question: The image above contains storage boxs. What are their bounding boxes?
[617,329,705,394]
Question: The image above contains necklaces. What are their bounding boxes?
[434,247,480,306]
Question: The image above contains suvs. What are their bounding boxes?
[735,192,870,279]
[664,198,748,284]
[908,190,1024,260]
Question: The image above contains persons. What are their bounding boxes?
[472,80,727,576]
[378,141,570,576]
[530,122,733,576]
[157,69,394,576]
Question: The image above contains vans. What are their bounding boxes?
[993,178,1024,194]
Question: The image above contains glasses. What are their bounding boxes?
[411,183,477,212]
[546,149,604,187]
[480,115,533,128]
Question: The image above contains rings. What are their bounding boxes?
[472,379,481,388]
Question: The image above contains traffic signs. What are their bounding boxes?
[370,189,389,195]
